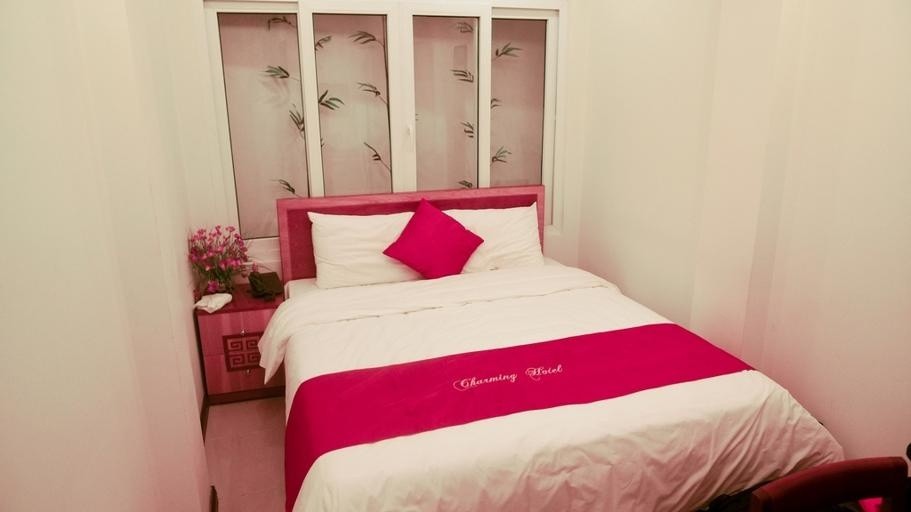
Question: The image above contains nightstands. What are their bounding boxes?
[195,282,286,405]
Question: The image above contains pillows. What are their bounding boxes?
[306,197,543,290]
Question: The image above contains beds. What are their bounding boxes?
[276,184,801,511]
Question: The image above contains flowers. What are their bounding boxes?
[186,223,249,292]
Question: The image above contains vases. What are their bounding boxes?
[215,278,227,292]
